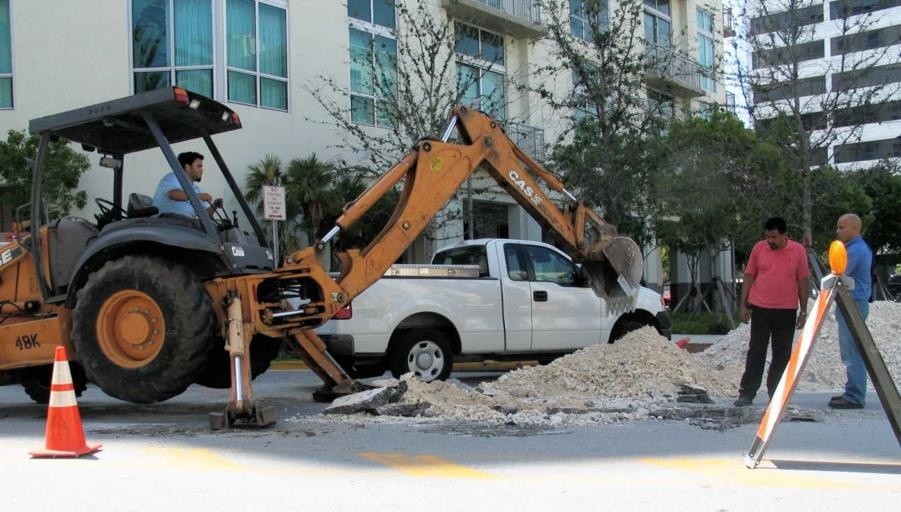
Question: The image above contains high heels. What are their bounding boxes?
[27,346,103,458]
[676,336,691,348]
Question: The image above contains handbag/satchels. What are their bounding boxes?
[800,311,809,317]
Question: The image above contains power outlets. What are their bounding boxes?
[299,235,674,370]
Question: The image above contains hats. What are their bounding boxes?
[662,282,672,304]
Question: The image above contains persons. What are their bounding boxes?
[148,152,226,221]
[828,213,875,410]
[731,217,812,408]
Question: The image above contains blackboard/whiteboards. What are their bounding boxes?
[1,90,645,430]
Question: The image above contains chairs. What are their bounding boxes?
[735,397,752,405]
[827,394,863,408]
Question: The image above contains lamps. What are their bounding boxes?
[127,193,159,216]
